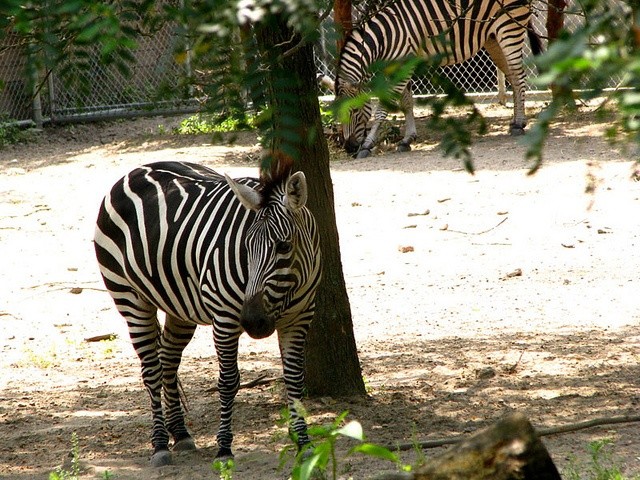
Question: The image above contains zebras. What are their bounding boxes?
[94,150,327,475]
[315,0,547,159]
